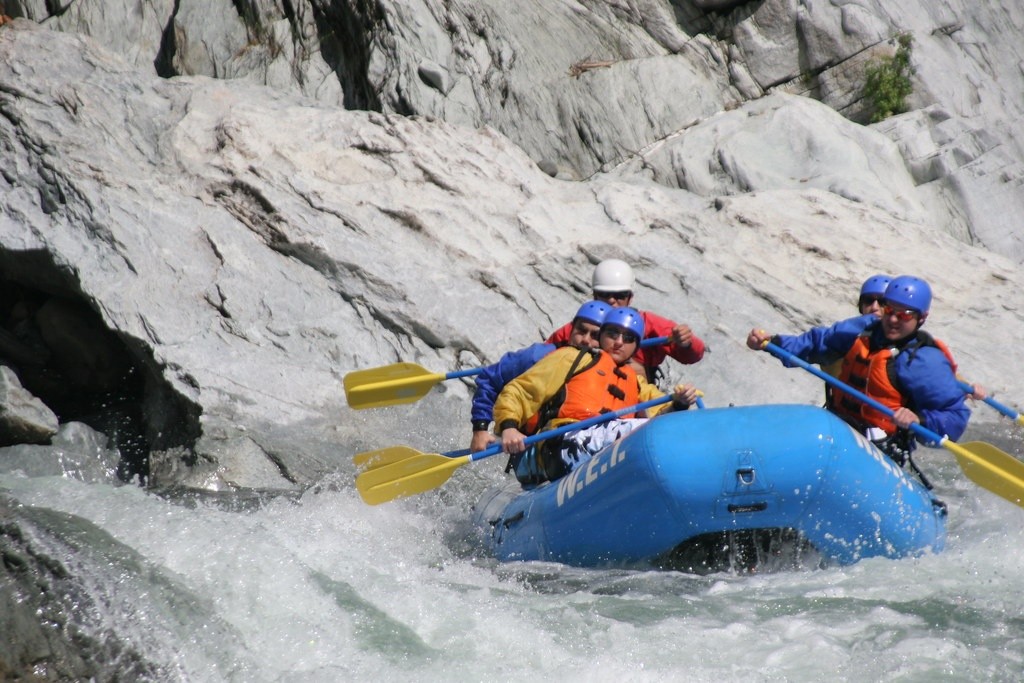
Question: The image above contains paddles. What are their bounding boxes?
[348,443,500,469]
[764,340,1024,510]
[358,392,675,505]
[955,375,1024,424]
[345,337,673,413]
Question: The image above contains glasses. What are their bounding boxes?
[604,327,636,343]
[863,294,887,306]
[883,304,918,321]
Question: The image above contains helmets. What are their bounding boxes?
[861,274,893,295]
[600,307,644,348]
[592,259,635,297]
[573,300,613,327]
[884,275,931,315]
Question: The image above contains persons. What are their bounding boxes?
[747,276,970,464]
[819,274,987,417]
[470,301,613,453]
[492,307,697,489]
[544,258,704,390]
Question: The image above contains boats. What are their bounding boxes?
[470,403,948,573]
